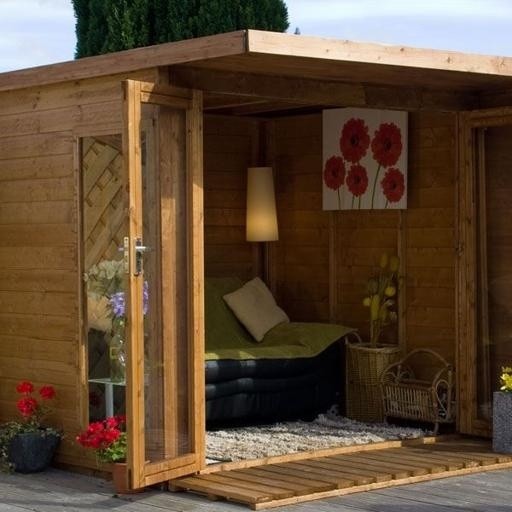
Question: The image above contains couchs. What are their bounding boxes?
[202,274,358,430]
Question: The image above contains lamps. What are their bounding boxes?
[245,165,280,283]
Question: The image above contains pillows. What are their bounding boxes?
[221,277,292,343]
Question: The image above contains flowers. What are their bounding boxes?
[362,252,401,347]
[1,379,57,475]
[75,413,128,465]
[497,365,511,391]
[81,250,151,329]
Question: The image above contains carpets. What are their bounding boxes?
[206,405,432,465]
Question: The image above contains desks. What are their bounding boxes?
[83,374,156,417]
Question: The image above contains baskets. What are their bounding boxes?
[344,332,402,418]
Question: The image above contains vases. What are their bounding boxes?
[492,391,512,455]
[110,459,151,495]
[5,432,60,473]
[108,335,125,383]
